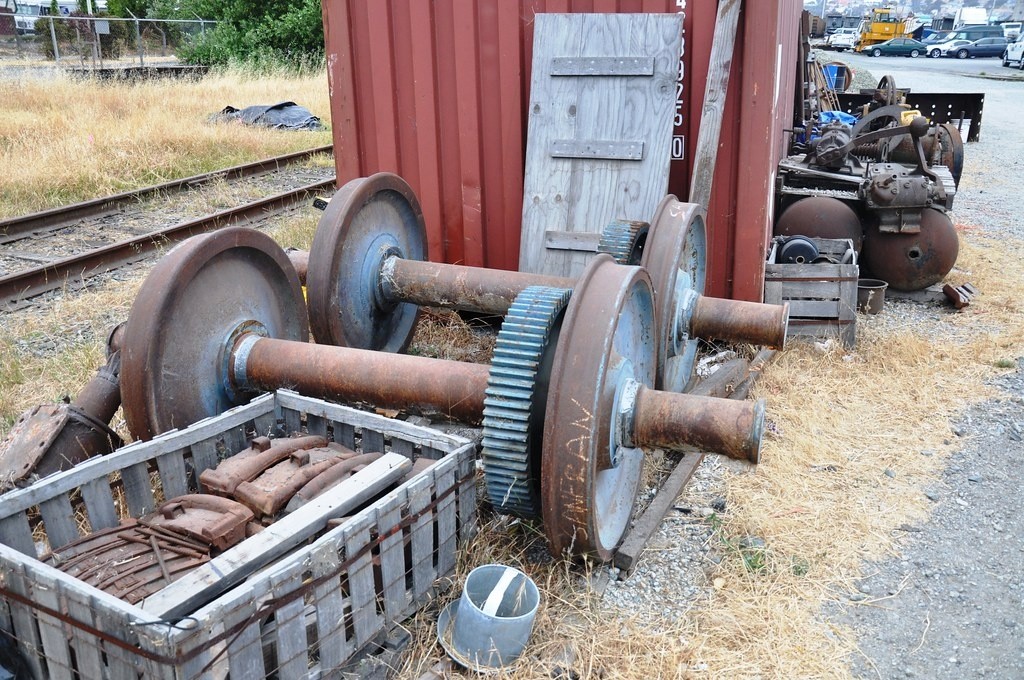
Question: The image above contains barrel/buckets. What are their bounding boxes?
[453,565,540,668]
[857,279,889,315]
[821,65,837,89]
[835,65,846,92]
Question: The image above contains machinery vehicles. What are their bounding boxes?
[856,6,915,53]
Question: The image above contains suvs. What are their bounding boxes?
[936,25,1004,46]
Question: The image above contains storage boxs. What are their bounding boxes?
[764,234,860,350]
[0,388,478,680]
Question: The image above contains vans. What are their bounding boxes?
[922,29,955,45]
[952,5,988,30]
[1000,22,1024,42]
[14,2,70,35]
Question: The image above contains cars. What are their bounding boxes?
[861,37,928,58]
[1003,30,1024,70]
[926,40,976,59]
[947,36,1008,60]
[822,28,858,52]
